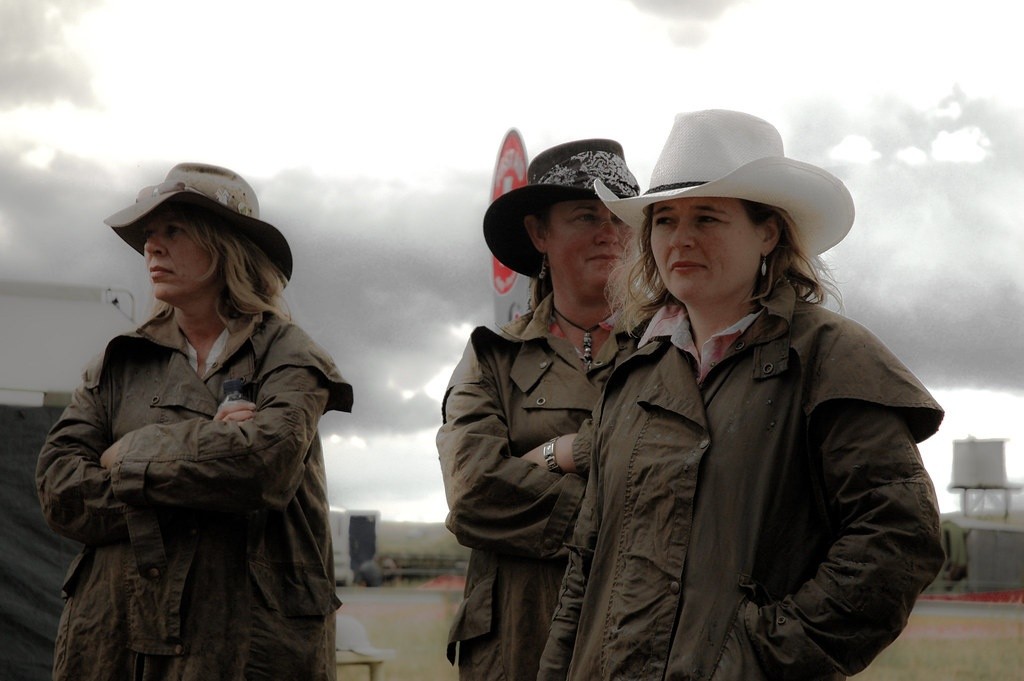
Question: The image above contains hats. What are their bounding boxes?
[592,108,855,257]
[482,139,640,279]
[103,162,293,293]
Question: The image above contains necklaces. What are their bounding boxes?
[552,297,612,364]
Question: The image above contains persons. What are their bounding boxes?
[538,108,944,681]
[435,139,640,680]
[36,163,354,680]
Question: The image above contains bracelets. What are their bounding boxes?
[544,436,562,473]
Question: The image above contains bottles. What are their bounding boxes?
[221,378,269,528]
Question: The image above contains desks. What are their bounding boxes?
[335,649,388,681]
